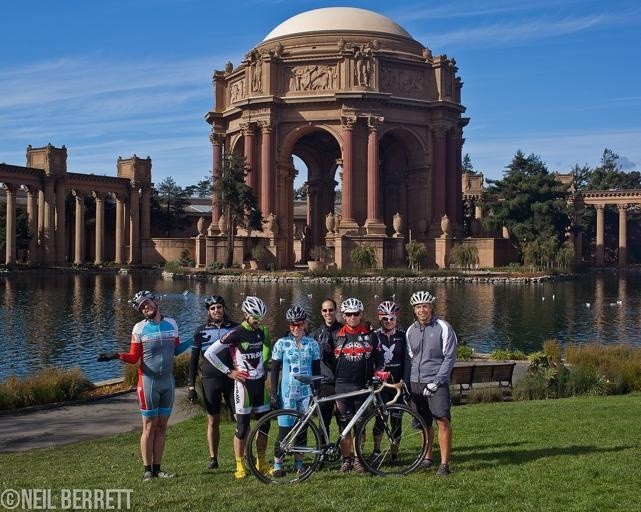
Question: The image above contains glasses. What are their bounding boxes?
[323,309,333,313]
[249,317,267,320]
[289,323,304,328]
[345,313,360,317]
[210,306,223,310]
[382,318,395,321]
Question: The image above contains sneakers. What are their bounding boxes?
[232,457,305,480]
[154,471,175,478]
[339,459,351,472]
[208,462,219,469]
[142,471,153,483]
[419,460,432,469]
[315,462,324,473]
[435,464,450,477]
[352,458,365,473]
[365,449,379,467]
[388,455,398,466]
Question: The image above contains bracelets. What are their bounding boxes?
[226,369,231,375]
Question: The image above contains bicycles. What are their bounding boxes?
[244,365,432,487]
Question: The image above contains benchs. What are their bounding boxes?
[448,363,518,398]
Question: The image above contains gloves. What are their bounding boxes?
[422,382,440,400]
[97,352,117,362]
[270,396,281,409]
[187,387,198,403]
[193,332,211,344]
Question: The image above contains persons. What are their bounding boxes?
[332,298,386,473]
[96,290,211,483]
[269,306,321,477]
[313,299,345,459]
[187,295,256,469]
[404,291,457,476]
[203,296,274,480]
[366,300,406,466]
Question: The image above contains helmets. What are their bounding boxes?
[240,296,267,317]
[204,295,226,310]
[409,291,437,307]
[339,297,365,314]
[130,290,153,311]
[377,301,401,317]
[285,307,307,322]
